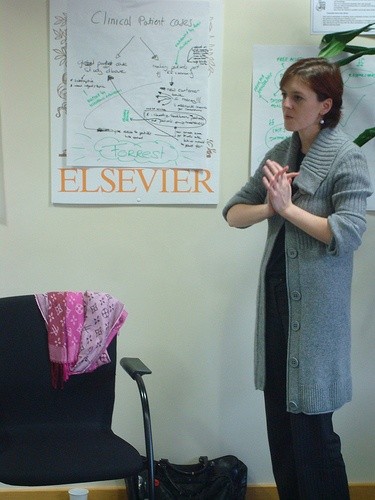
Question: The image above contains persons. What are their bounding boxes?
[222,56,373,499]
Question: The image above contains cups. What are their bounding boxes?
[68,488,89,500]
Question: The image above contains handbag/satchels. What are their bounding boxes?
[155,455,248,500]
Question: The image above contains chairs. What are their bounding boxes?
[0,292,155,499]
